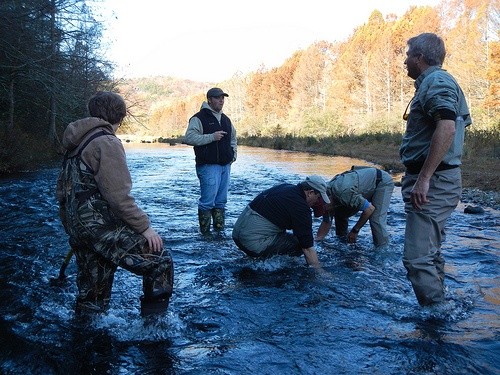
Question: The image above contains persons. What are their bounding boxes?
[183,88,238,235]
[312,165,395,249]
[56,91,174,330]
[400,32,471,310]
[230,175,330,271]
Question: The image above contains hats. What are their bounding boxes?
[207,88,229,99]
[311,195,323,217]
[306,175,330,205]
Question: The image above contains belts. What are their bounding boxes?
[376,168,382,187]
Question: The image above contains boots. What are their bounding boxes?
[198,209,214,240]
[138,294,169,317]
[212,208,227,241]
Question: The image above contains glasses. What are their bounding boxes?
[118,118,121,127]
[403,94,416,121]
